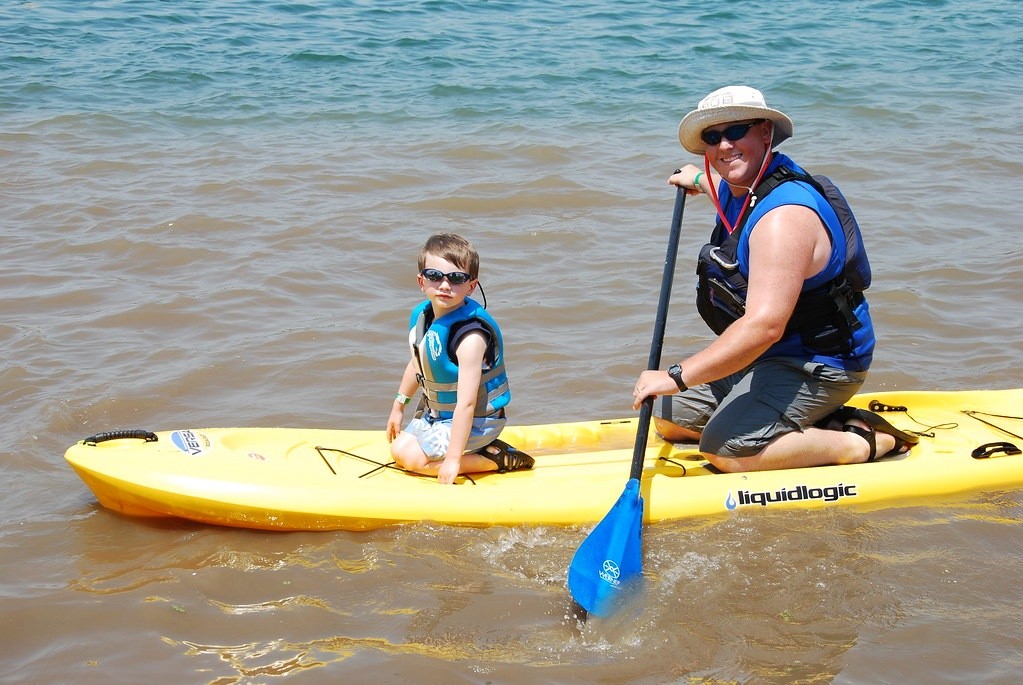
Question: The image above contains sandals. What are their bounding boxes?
[478,439,535,473]
[843,409,919,464]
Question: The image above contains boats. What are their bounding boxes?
[62,387,1022,536]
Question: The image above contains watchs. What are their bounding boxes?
[668,363,688,392]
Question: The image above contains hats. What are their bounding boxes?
[678,85,793,155]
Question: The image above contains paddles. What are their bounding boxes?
[564,185,689,620]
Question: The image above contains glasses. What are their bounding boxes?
[421,268,470,284]
[701,119,763,145]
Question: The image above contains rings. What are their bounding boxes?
[637,388,641,393]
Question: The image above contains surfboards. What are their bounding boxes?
[60,382,1023,535]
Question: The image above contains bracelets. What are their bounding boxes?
[695,172,705,193]
[395,394,410,405]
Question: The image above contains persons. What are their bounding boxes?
[387,233,536,484]
[631,85,918,473]
